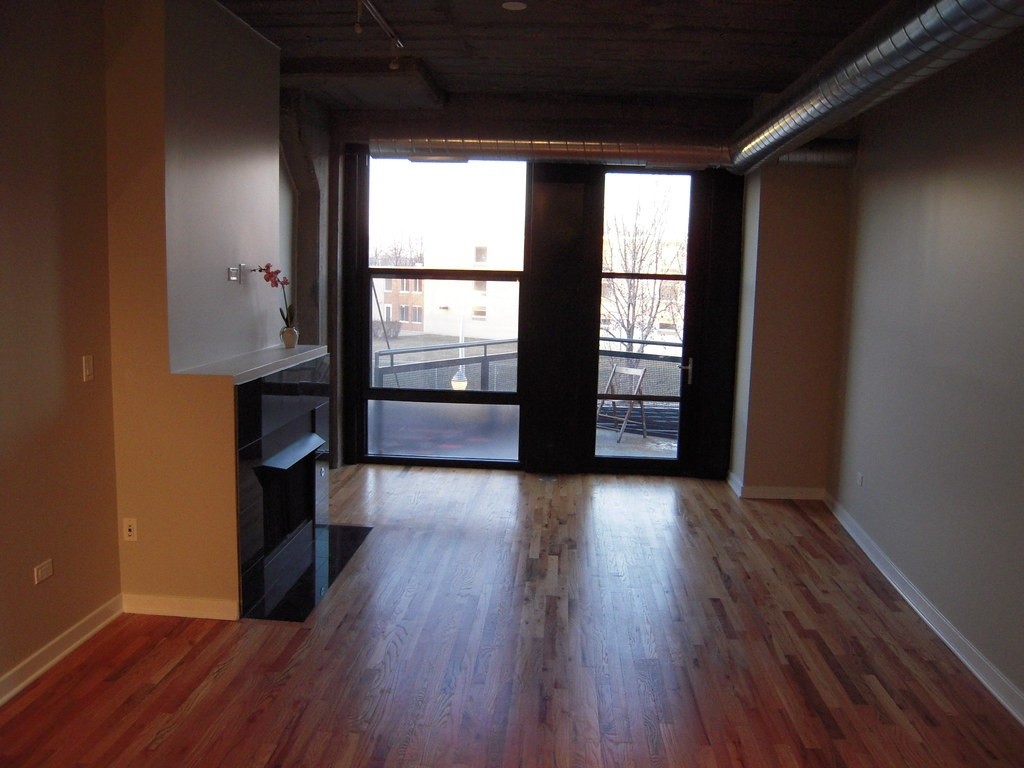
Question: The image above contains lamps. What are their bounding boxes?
[390,38,401,71]
[354,1,365,34]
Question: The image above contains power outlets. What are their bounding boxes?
[230,268,240,280]
[33,560,55,583]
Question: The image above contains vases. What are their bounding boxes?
[283,328,300,348]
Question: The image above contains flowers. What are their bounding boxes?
[251,264,298,344]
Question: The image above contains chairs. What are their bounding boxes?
[595,364,648,441]
